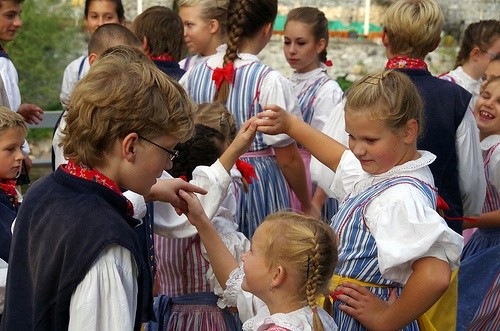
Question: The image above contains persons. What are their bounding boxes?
[1,0,500,331]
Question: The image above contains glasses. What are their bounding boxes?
[482,48,500,61]
[122,132,179,161]
